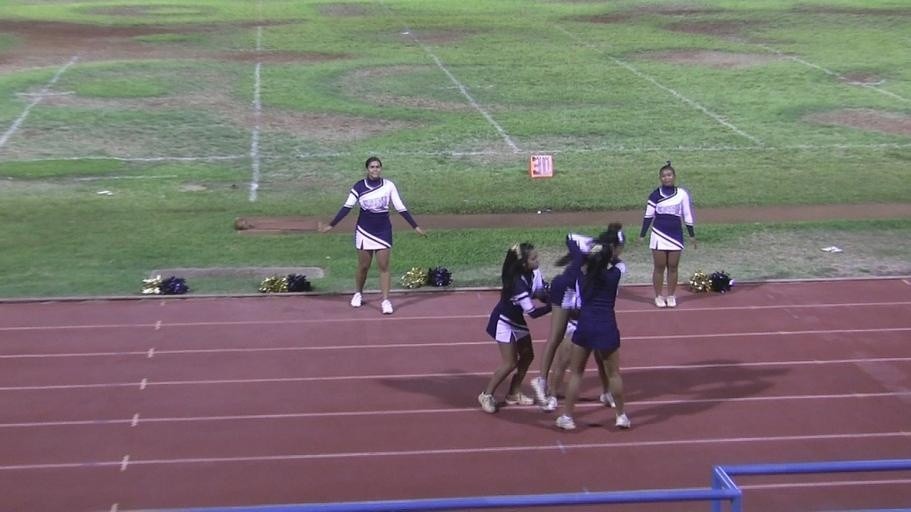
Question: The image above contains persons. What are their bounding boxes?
[320,157,427,314]
[478,223,631,430]
[635,166,697,308]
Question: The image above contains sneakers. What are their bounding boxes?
[600,392,616,408]
[655,295,666,308]
[504,392,534,405]
[381,299,394,314]
[616,412,631,429]
[555,413,576,431]
[530,376,558,411]
[666,295,677,308]
[350,292,362,307]
[478,392,496,414]
